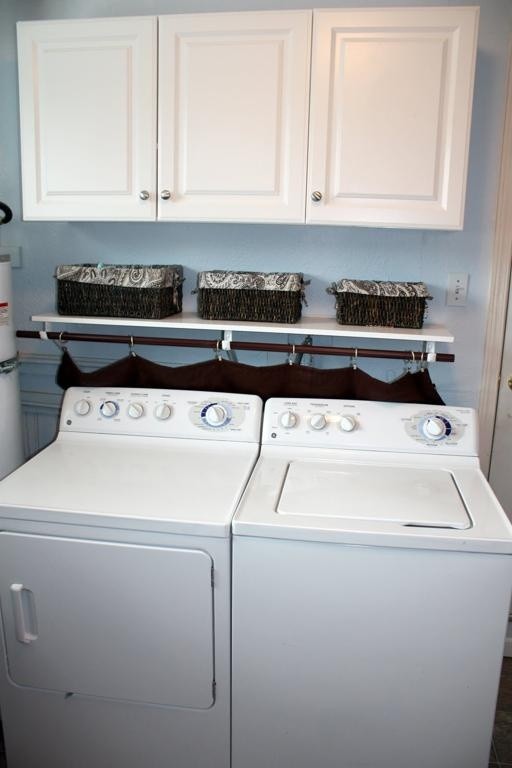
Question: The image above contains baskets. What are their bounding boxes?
[197,270,303,324]
[57,263,183,320]
[336,278,428,329]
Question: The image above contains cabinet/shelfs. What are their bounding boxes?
[309,2,484,236]
[12,3,316,226]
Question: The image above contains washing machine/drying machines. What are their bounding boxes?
[2,385,264,768]
[229,395,512,768]
